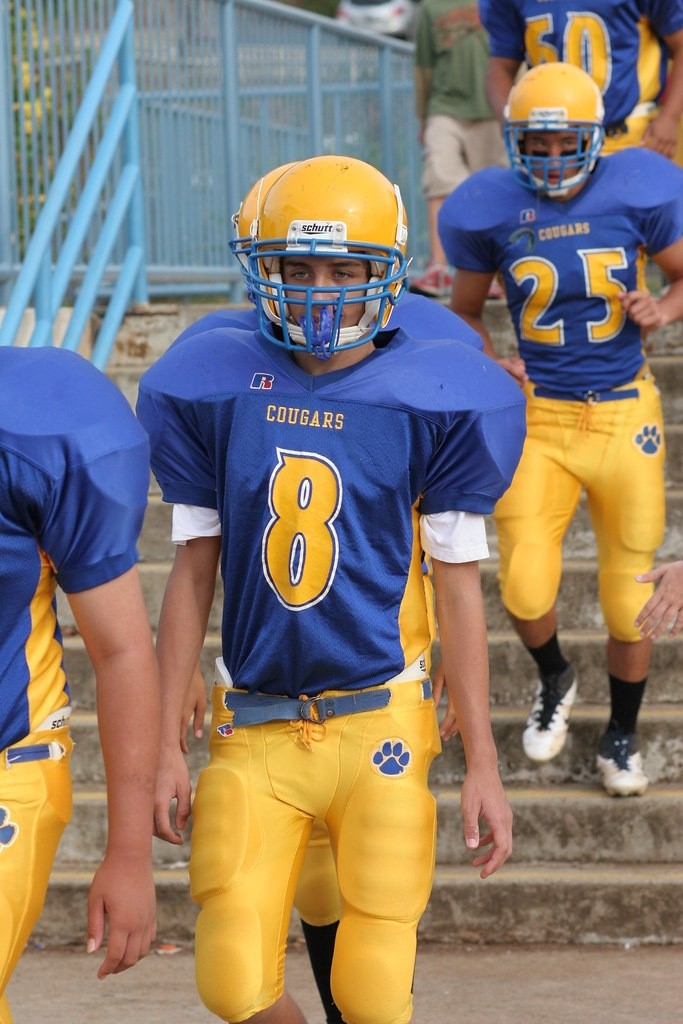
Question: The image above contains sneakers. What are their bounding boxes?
[487,281,505,300]
[596,729,650,798]
[410,271,455,297]
[520,661,577,763]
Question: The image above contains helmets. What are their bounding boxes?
[231,154,410,362]
[502,60,605,189]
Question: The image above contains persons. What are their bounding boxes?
[437,61,683,802]
[476,0,682,186]
[134,154,529,1024]
[0,342,161,1024]
[406,0,510,300]
[131,159,488,1021]
